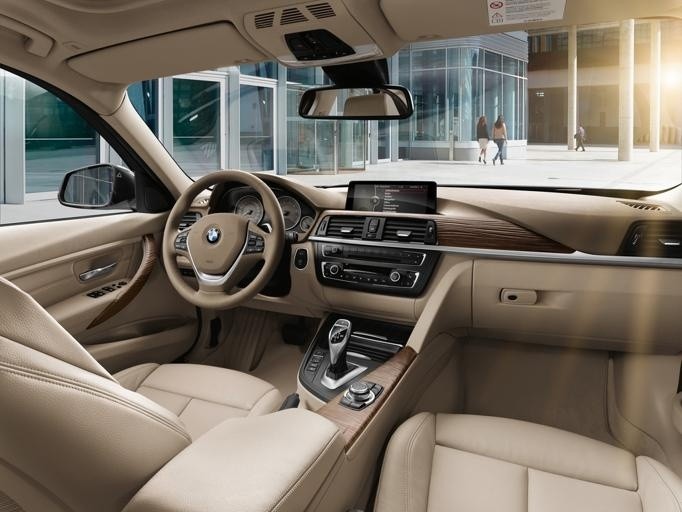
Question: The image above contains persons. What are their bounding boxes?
[477,116,489,164]
[575,123,586,151]
[492,115,507,166]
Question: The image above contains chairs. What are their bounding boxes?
[343,94,399,117]
[372,408,680,511]
[0,274,278,511]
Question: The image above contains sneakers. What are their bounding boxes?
[501,163,504,165]
[478,156,481,162]
[483,160,486,164]
[493,160,495,165]
